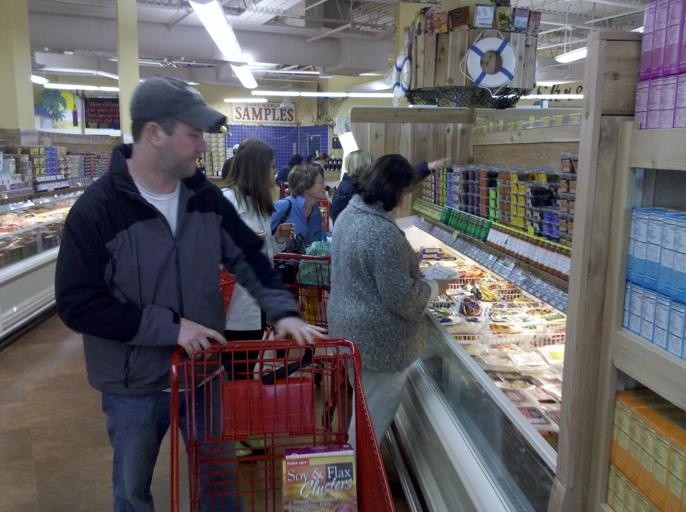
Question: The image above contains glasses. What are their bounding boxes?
[272,165,276,169]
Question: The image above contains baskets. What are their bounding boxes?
[427,278,566,349]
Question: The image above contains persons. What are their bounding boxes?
[274,154,304,200]
[311,153,330,184]
[327,147,452,230]
[211,137,297,382]
[220,142,239,181]
[51,70,329,512]
[269,162,332,302]
[322,150,451,485]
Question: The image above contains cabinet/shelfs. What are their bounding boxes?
[0,29,686,512]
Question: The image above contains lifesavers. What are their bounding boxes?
[467,37,515,88]
[391,55,411,99]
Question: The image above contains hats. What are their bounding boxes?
[233,144,239,152]
[130,75,226,133]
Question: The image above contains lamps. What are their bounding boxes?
[31,0,644,105]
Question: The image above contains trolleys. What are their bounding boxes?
[258,252,337,427]
[278,182,329,233]
[171,338,398,512]
